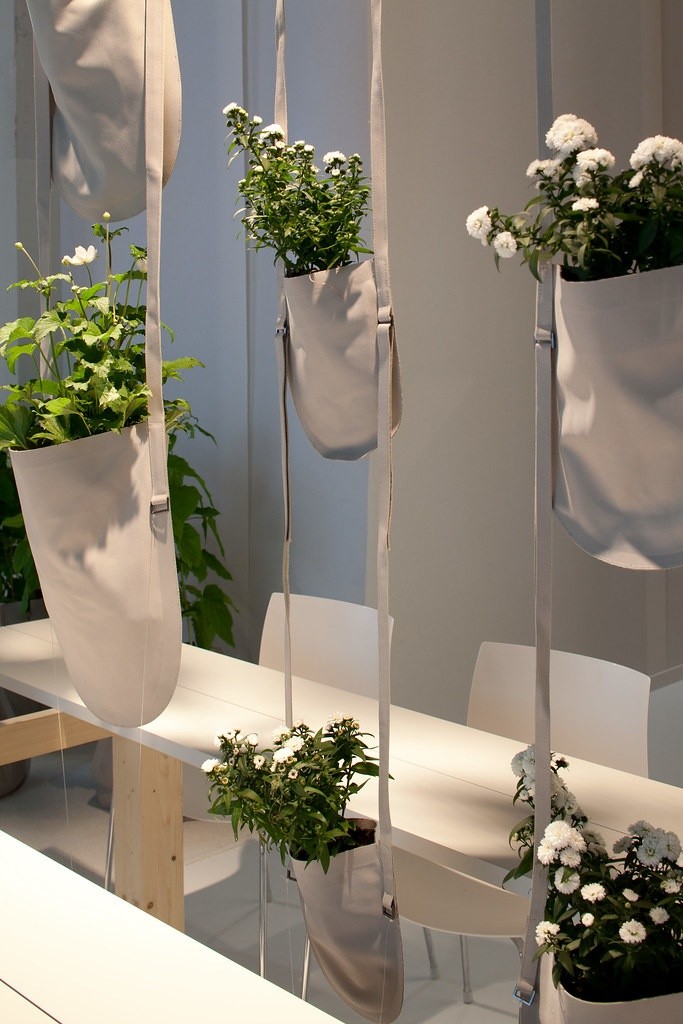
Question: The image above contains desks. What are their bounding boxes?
[0,620,683,1023]
[0,830,338,1024]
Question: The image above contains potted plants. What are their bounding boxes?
[2,417,243,796]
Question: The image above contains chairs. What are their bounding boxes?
[300,642,649,1000]
[104,594,393,977]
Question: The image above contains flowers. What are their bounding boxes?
[223,102,376,277]
[1,214,208,446]
[501,746,682,1002]
[466,113,682,279]
[200,719,393,875]
